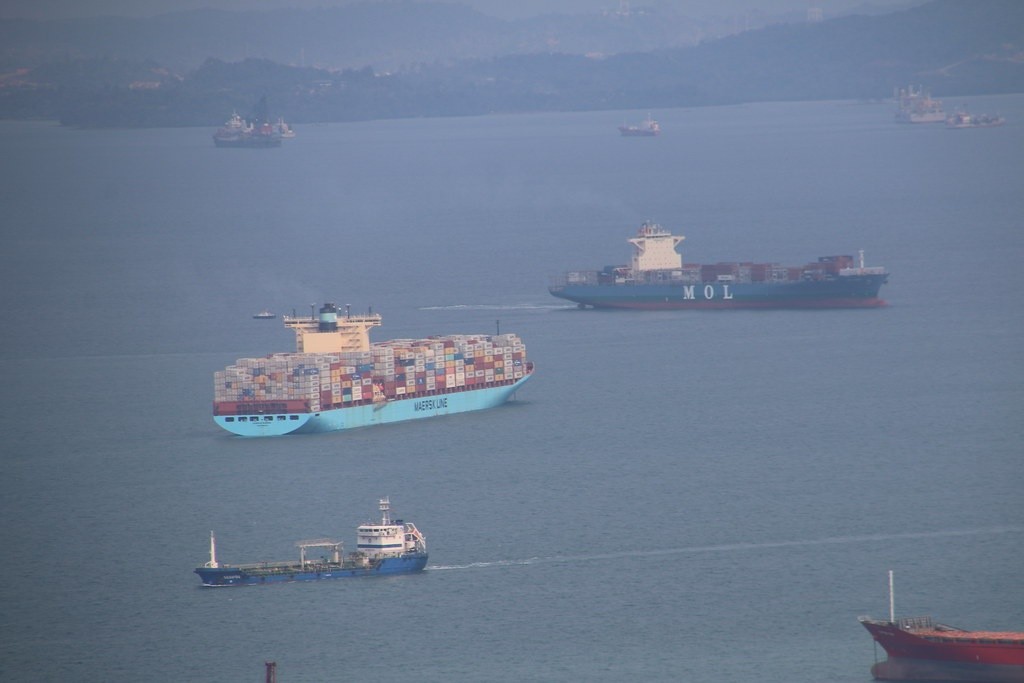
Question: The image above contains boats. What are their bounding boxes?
[194,495,432,588]
[213,108,295,149]
[617,113,662,136]
[215,299,535,439]
[253,312,275,319]
[858,567,1024,683]
[897,84,1009,128]
[547,217,891,309]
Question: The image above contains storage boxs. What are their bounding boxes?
[213,332,528,413]
